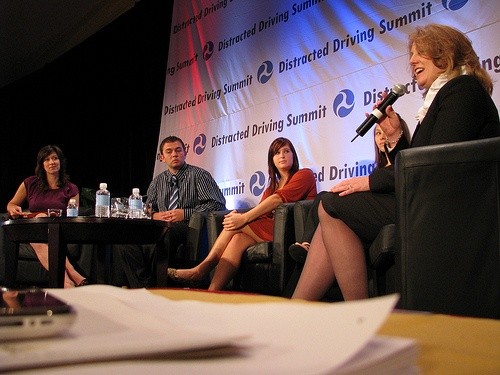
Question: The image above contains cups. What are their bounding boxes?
[48,208,62,217]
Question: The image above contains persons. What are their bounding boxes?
[289,24,500,302]
[165,137,317,290]
[111,136,226,289]
[7,146,87,288]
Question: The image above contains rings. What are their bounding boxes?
[348,185,352,189]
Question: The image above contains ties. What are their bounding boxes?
[169,175,178,210]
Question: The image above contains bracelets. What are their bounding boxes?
[385,131,404,143]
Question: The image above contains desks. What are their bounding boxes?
[0,216,169,289]
[147,289,500,375]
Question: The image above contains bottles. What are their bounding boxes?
[95,183,110,217]
[67,198,78,217]
[129,188,142,218]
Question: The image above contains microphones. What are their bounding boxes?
[351,83,406,142]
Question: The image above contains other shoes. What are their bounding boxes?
[290,242,309,263]
[167,268,205,286]
[78,278,88,286]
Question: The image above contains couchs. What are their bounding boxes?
[206,202,301,297]
[116,196,205,289]
[294,137,500,321]
[1,209,108,287]
